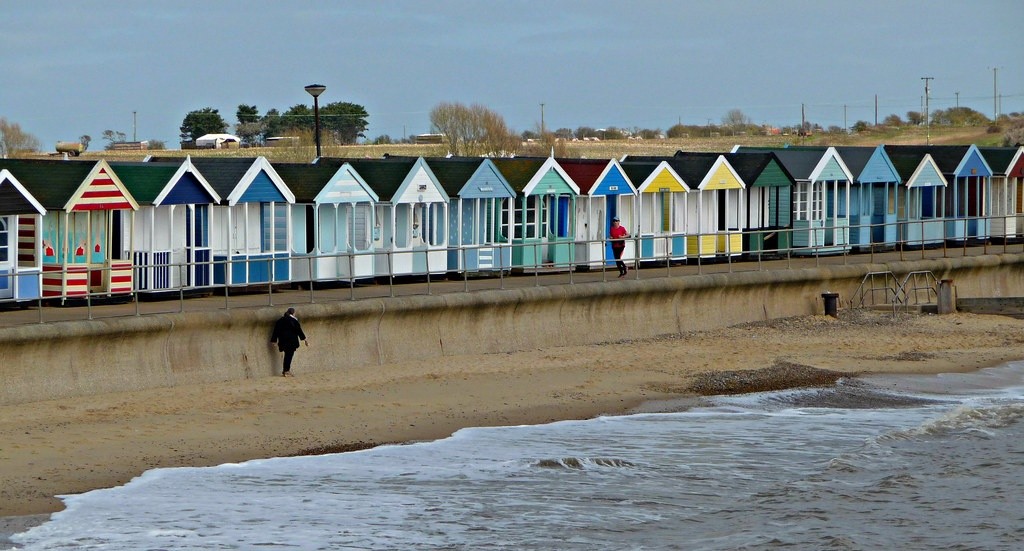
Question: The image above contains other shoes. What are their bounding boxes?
[618,267,628,278]
[282,372,295,377]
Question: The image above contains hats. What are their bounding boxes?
[613,217,620,221]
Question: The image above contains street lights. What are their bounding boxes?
[305,84,327,157]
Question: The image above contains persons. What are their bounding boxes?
[609,216,631,278]
[270,307,310,378]
